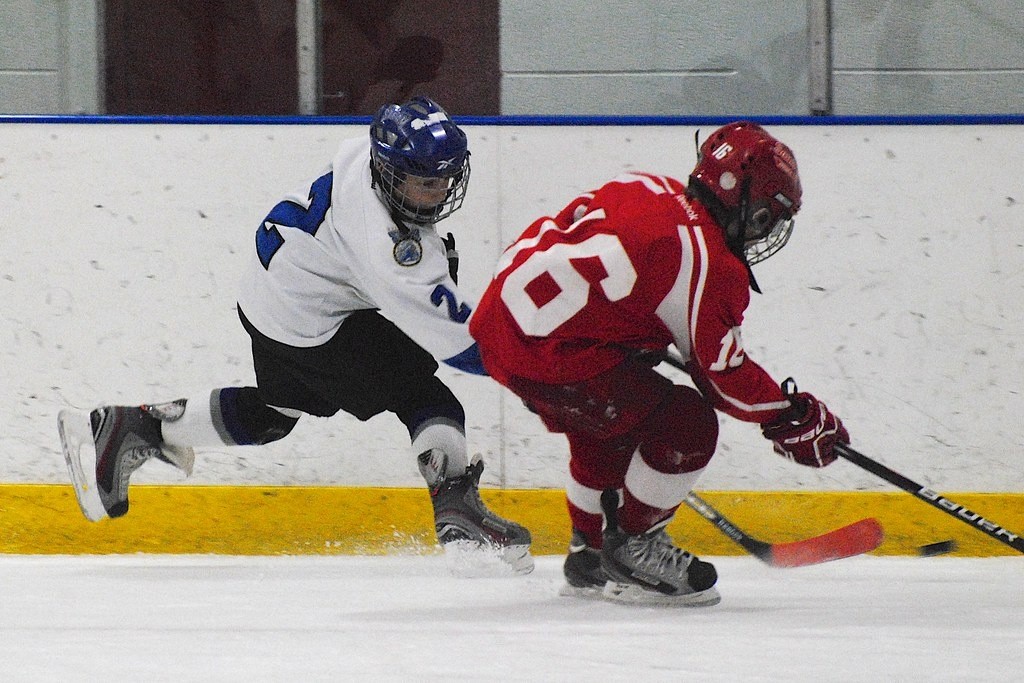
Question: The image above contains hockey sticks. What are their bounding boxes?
[666,344,1024,551]
[683,490,884,564]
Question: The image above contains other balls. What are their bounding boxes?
[920,540,954,556]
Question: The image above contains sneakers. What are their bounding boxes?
[418,448,535,579]
[558,526,614,601]
[599,488,722,608]
[58,399,195,523]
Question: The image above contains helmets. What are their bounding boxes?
[370,96,469,192]
[689,120,803,222]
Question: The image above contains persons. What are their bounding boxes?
[472,120,854,607]
[59,99,534,577]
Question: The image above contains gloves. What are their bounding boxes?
[762,376,851,467]
